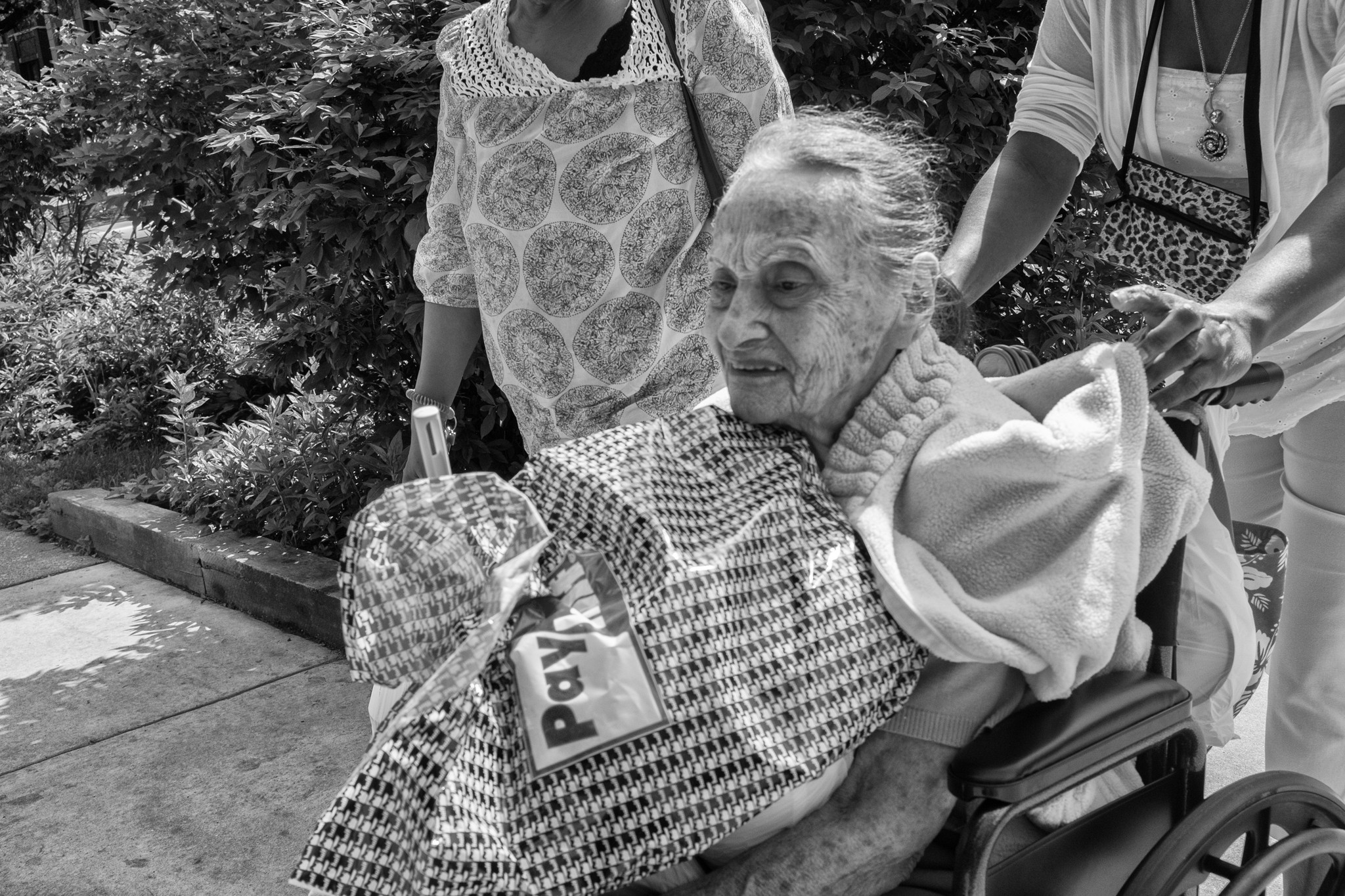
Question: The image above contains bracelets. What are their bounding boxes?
[406,389,455,420]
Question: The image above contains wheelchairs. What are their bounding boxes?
[883,314,1345,896]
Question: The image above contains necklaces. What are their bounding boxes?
[1190,0,1251,161]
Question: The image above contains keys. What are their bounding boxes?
[444,426,456,455]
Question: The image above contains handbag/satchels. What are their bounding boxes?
[1171,399,1290,725]
[1095,152,1273,307]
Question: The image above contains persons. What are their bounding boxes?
[401,0,791,484]
[671,113,1044,896]
[942,0,1344,813]
